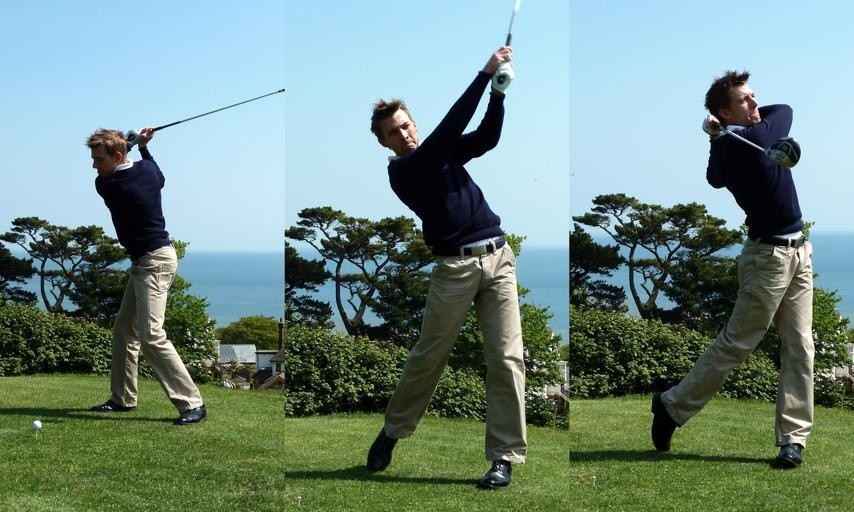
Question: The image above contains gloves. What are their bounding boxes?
[126,128,142,147]
[491,63,514,93]
[702,113,721,136]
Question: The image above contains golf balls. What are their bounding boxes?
[34,420,42,429]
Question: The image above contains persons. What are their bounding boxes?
[368,46,527,489]
[85,128,206,424]
[652,70,815,466]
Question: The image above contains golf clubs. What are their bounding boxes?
[718,125,800,167]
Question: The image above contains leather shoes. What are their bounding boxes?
[776,444,802,467]
[174,406,207,425]
[91,399,136,411]
[478,460,512,489]
[652,392,677,451]
[367,428,397,471]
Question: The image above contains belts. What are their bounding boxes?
[432,238,505,257]
[750,232,805,248]
